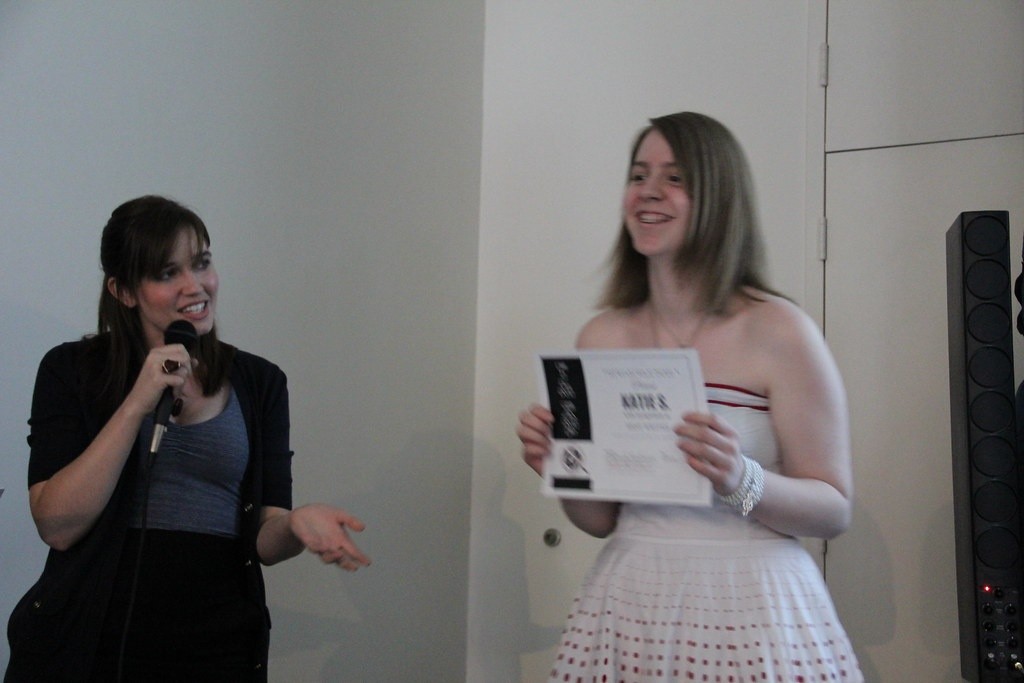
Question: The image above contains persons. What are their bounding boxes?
[3,195,371,683]
[515,111,865,683]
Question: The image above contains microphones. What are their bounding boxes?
[148,320,198,454]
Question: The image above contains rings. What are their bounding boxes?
[162,359,181,374]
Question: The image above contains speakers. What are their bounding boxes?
[946,210,1024,683]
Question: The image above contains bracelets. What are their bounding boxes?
[718,455,764,518]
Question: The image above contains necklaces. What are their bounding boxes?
[653,305,706,348]
